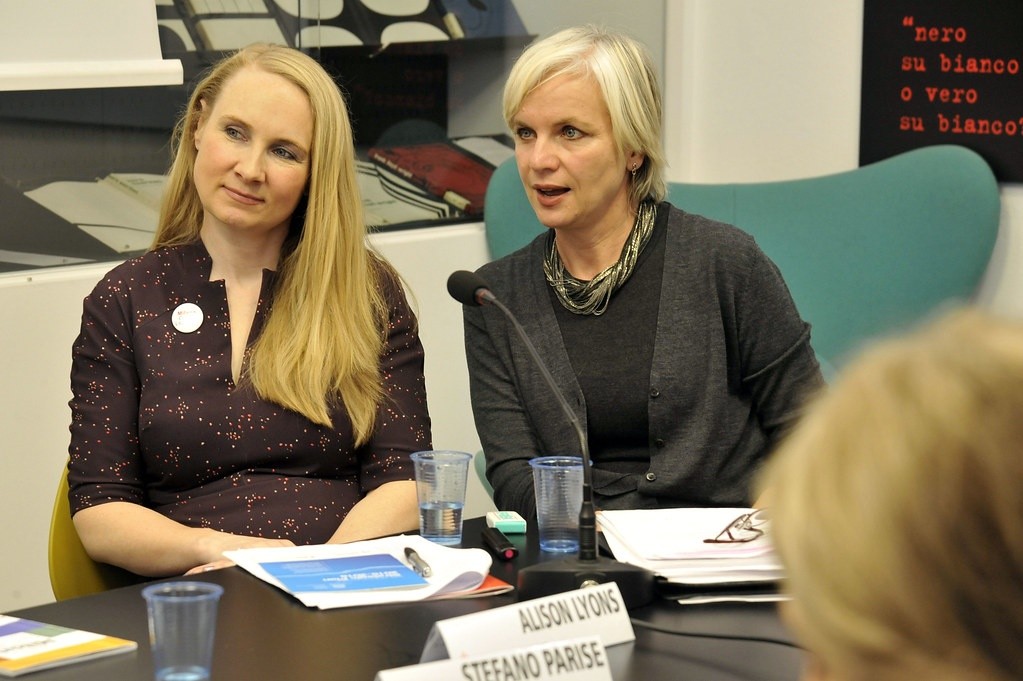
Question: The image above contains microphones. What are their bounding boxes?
[447,269,655,609]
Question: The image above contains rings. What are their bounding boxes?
[204,566,213,572]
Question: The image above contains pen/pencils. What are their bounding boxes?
[404,547,431,578]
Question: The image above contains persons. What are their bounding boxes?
[746,300,1023,681]
[459,25,829,521]
[68,45,436,585]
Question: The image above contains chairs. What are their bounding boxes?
[486,146,1002,399]
[47,466,119,602]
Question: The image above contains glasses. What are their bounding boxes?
[703,508,770,544]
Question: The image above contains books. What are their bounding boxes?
[0,613,139,678]
[261,547,429,594]
[598,503,790,608]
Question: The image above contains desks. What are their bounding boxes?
[0,510,819,681]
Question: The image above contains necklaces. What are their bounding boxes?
[542,195,657,316]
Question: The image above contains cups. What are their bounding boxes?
[141,582,225,681]
[528,455,593,553]
[409,451,473,546]
